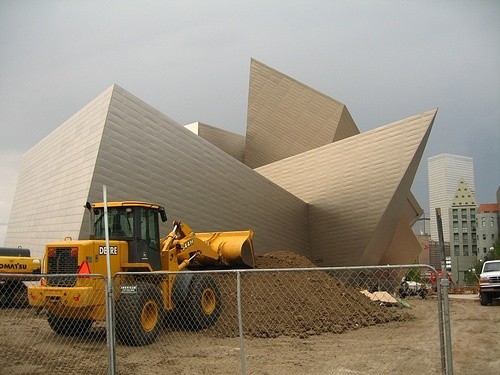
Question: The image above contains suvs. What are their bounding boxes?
[479,260,500,305]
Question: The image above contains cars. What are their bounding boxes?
[400,276,426,299]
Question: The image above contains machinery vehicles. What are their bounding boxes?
[1,245,42,308]
[41,199,255,345]
